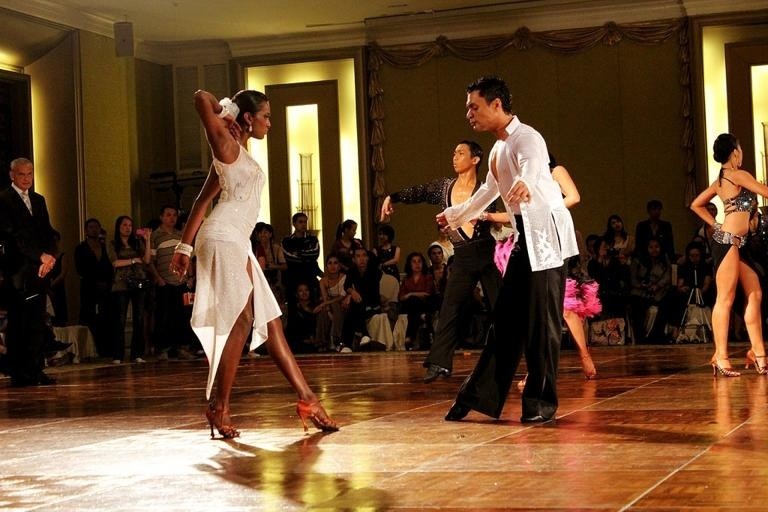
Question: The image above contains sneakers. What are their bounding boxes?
[157,351,168,361]
[135,358,145,363]
[360,336,370,346]
[112,360,120,363]
[341,347,352,353]
[649,328,674,345]
[178,351,197,360]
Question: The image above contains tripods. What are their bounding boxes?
[675,269,713,343]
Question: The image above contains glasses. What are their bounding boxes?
[411,259,422,264]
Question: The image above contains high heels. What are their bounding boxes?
[206,404,239,438]
[297,401,340,432]
[581,354,596,378]
[745,351,768,375]
[712,355,740,376]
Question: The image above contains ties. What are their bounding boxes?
[20,192,32,215]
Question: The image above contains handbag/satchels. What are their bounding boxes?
[125,265,154,291]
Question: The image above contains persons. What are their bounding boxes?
[676,194,768,345]
[635,199,675,265]
[398,252,433,351]
[0,159,56,386]
[332,220,362,272]
[255,224,287,287]
[35,319,73,370]
[283,213,319,279]
[379,141,501,383]
[312,253,354,353]
[626,237,686,345]
[440,254,483,348]
[696,203,717,263]
[169,90,340,438]
[582,234,599,273]
[372,224,401,281]
[285,282,317,354]
[436,76,579,424]
[691,134,768,378]
[478,153,601,387]
[677,243,713,307]
[74,218,114,363]
[250,222,265,255]
[97,228,106,243]
[336,248,380,353]
[599,215,634,254]
[150,206,199,361]
[427,245,446,293]
[47,231,68,327]
[429,226,454,265]
[108,216,152,364]
[588,236,631,306]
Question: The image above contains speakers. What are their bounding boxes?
[113,21,135,58]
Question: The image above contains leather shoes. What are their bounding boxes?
[422,365,451,383]
[52,340,72,352]
[38,371,57,384]
[520,414,554,423]
[445,399,472,420]
